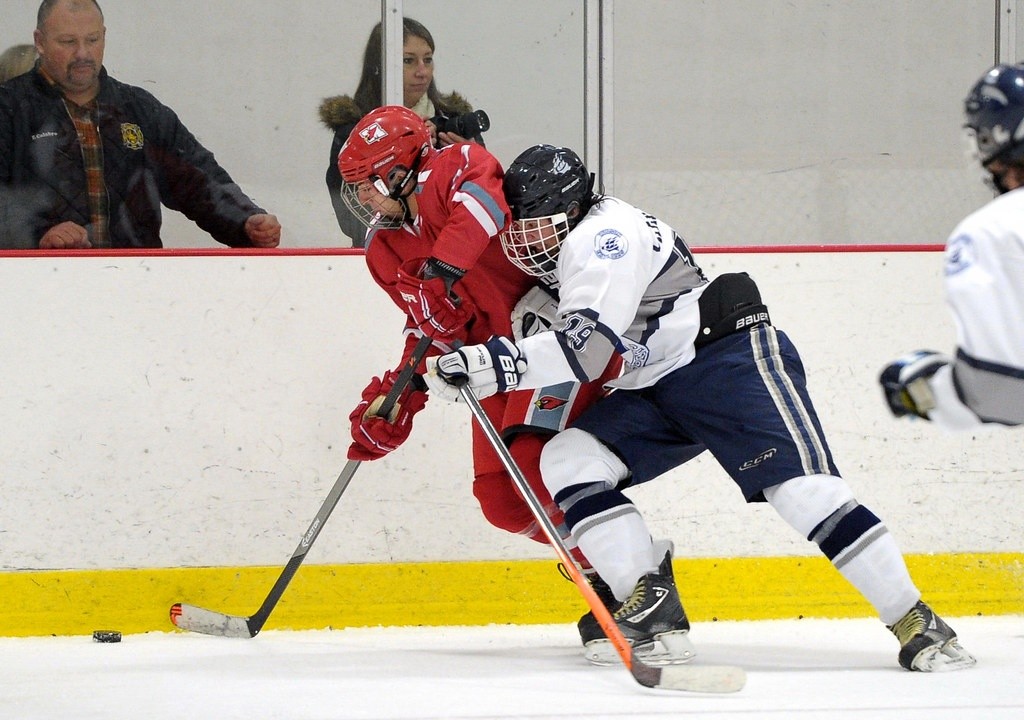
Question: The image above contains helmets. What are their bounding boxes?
[962,64,1024,168]
[339,105,432,197]
[502,144,595,229]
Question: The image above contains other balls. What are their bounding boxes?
[92,629,122,643]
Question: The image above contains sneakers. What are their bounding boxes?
[557,562,623,623]
[575,538,697,666]
[885,599,978,673]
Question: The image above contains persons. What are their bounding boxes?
[0,0,281,250]
[422,143,977,674]
[338,105,626,628]
[880,60,1024,427]
[326,18,486,250]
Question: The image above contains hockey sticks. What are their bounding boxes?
[436,368,748,694]
[169,333,434,639]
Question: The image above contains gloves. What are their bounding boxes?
[880,347,949,421]
[423,333,528,404]
[344,369,429,462]
[395,256,489,353]
